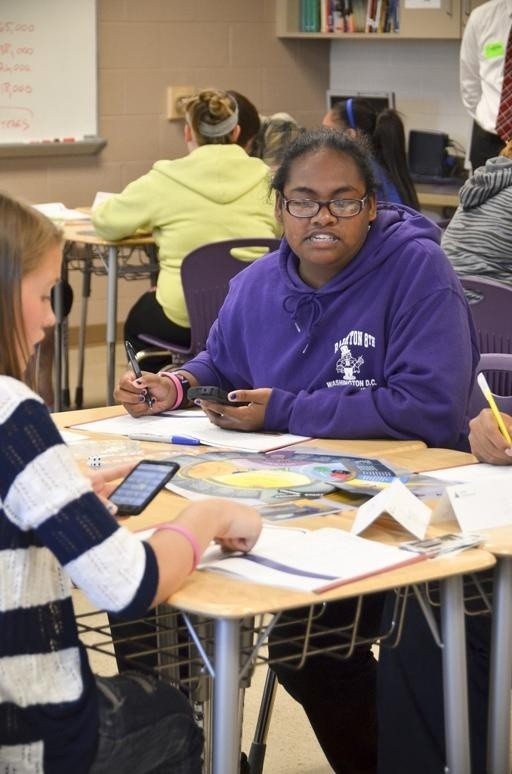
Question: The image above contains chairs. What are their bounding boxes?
[468,352,512,414]
[459,275,511,396]
[137,237,283,371]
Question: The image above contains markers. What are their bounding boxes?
[29,138,52,145]
[53,137,76,143]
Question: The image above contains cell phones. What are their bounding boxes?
[186,384,239,406]
[107,458,181,516]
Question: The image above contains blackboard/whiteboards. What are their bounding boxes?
[0,0,107,156]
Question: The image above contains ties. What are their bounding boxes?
[496,22,512,144]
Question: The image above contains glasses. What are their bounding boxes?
[279,191,369,220]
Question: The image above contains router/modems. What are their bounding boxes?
[407,127,450,176]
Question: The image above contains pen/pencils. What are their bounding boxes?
[128,432,200,446]
[477,371,512,444]
[124,340,153,410]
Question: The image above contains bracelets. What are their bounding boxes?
[174,373,191,409]
[159,372,183,411]
[154,523,201,575]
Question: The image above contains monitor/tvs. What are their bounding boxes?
[325,90,395,113]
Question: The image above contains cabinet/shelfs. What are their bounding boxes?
[276,0,489,40]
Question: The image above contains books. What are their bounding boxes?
[67,408,314,454]
[28,202,93,225]
[299,0,400,33]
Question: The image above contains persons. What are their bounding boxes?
[324,97,421,214]
[92,89,285,376]
[460,1,512,173]
[228,91,306,174]
[0,193,263,774]
[467,408,512,465]
[441,138,512,303]
[112,129,481,774]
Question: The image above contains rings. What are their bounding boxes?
[220,408,225,415]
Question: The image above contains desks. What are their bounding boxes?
[57,207,157,406]
[409,175,463,224]
[45,403,512,774]
